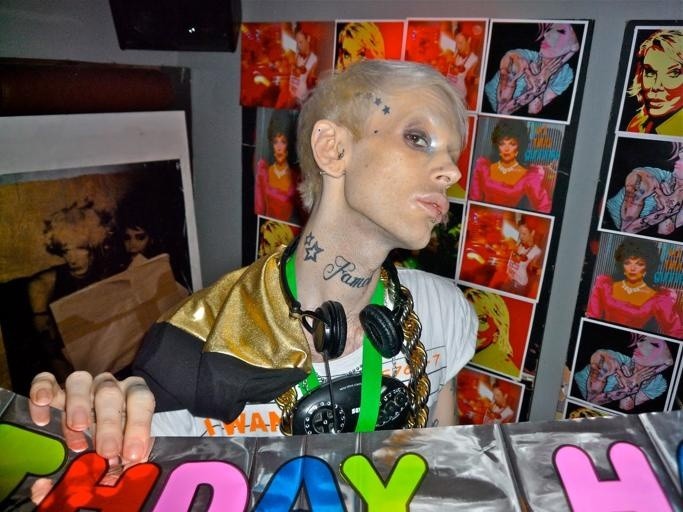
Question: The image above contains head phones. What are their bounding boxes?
[279,232,406,359]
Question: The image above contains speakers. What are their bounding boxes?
[108,0,241,51]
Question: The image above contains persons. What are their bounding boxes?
[24,60,478,466]
[40,200,115,323]
[112,205,161,274]
